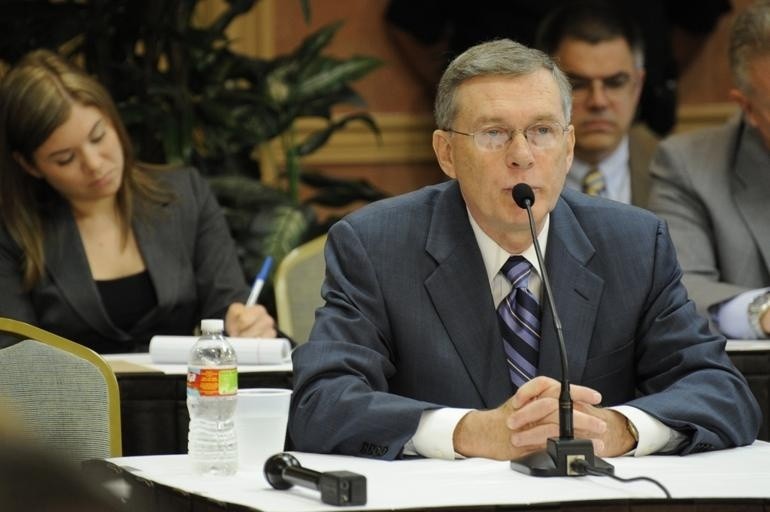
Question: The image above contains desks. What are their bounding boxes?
[99,439,769,511]
[101,338,769,454]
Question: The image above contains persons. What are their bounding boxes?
[0,48,280,396]
[290,38,763,462]
[644,4,770,340]
[539,6,665,209]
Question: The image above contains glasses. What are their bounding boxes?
[450,122,569,153]
[569,72,630,92]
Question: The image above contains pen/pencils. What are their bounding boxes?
[244,255,272,307]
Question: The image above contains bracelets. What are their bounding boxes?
[625,418,639,444]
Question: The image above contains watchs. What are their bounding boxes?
[749,289,769,340]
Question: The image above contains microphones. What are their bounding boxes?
[510,182,615,478]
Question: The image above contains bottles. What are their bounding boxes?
[184,317,243,474]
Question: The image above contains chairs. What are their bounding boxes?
[1,316,124,471]
[274,230,329,347]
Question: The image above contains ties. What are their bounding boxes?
[497,256,542,393]
[582,167,609,198]
